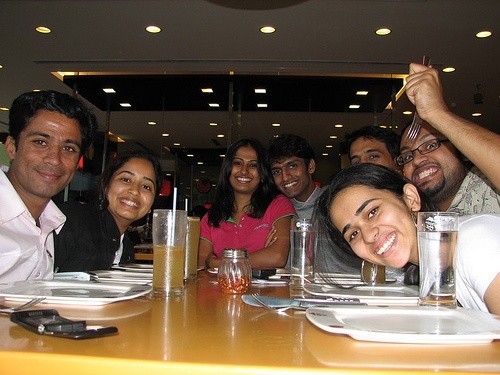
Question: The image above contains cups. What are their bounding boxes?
[152,209,187,298]
[292,218,317,289]
[417,210,459,311]
[361,260,385,288]
[185,216,200,283]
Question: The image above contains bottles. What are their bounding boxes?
[217,247,252,294]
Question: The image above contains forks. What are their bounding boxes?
[406,56,431,141]
[0,297,46,314]
[248,294,306,311]
[318,272,367,289]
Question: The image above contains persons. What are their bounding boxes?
[340,125,405,284]
[195,137,298,269]
[306,162,500,318]
[53,150,163,273]
[265,132,363,273]
[395,62,500,216]
[0,90,97,283]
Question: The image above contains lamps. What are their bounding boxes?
[474,84,483,104]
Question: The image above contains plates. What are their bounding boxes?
[91,270,153,285]
[302,283,419,299]
[111,263,153,272]
[0,278,153,306]
[306,307,500,344]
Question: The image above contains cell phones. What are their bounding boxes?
[10,309,118,339]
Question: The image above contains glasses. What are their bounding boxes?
[395,138,449,173]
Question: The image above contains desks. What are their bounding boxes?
[0,265,499,375]
[134,243,154,261]
